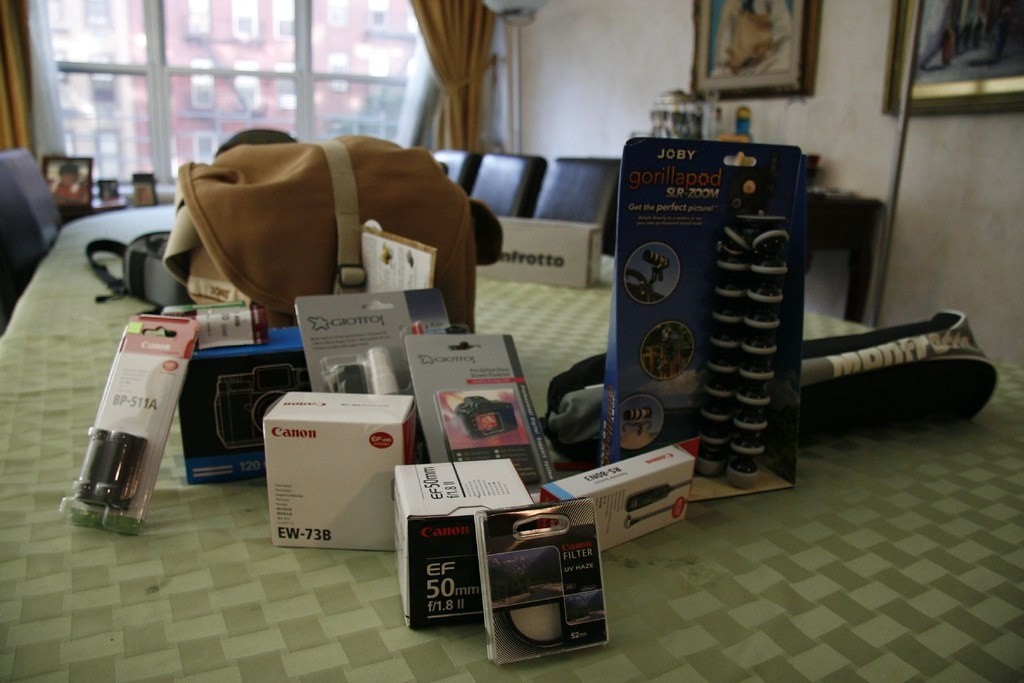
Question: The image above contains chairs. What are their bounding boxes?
[0,148,60,327]
[534,158,621,244]
[471,153,548,222]
[436,149,480,191]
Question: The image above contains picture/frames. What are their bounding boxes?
[45,158,97,211]
[689,0,821,100]
[883,0,1024,117]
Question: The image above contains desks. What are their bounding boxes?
[809,195,882,323]
[0,199,1024,683]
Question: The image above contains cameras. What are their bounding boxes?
[453,397,519,440]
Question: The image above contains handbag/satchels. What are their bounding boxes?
[85,232,195,314]
[163,130,503,334]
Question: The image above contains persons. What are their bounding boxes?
[52,164,89,205]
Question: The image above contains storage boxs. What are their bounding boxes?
[177,323,311,486]
[602,135,803,506]
[393,460,537,628]
[261,391,416,554]
[481,217,603,289]
[540,434,698,551]
